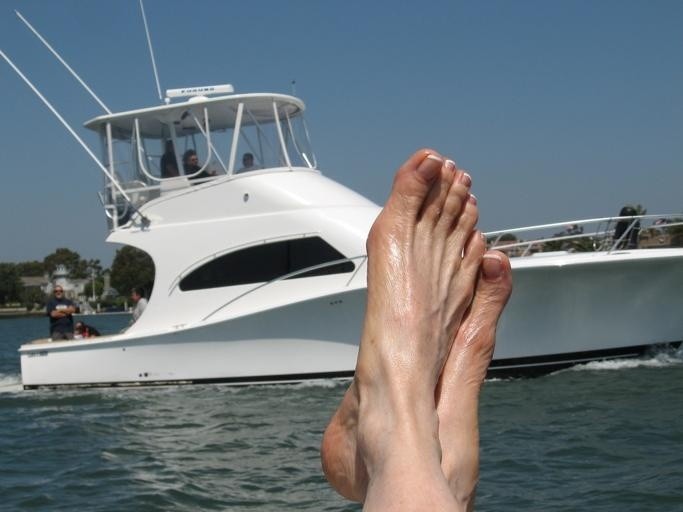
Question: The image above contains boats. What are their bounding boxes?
[0,4,683,391]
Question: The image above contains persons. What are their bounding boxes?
[234,154,263,173]
[47,285,101,341]
[128,285,148,321]
[161,140,179,179]
[322,149,515,511]
[183,150,217,185]
